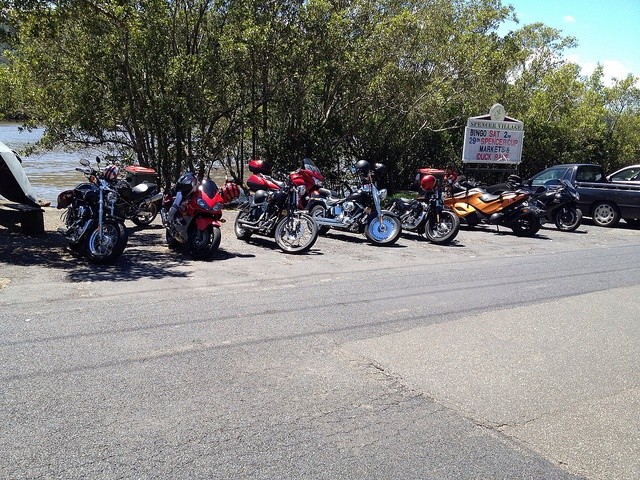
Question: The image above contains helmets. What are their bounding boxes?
[221,183,239,203]
[57,190,73,208]
[372,162,387,178]
[420,175,437,191]
[102,164,119,180]
[356,159,370,175]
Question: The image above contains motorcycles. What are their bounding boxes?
[440,174,545,236]
[96,156,162,226]
[506,175,583,232]
[306,159,402,246]
[56,159,136,264]
[379,167,460,245]
[160,175,228,257]
[234,158,325,254]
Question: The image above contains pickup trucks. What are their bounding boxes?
[485,163,640,229]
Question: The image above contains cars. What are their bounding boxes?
[606,165,640,183]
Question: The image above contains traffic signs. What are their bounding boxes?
[462,126,524,164]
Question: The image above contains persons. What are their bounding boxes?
[444,165,457,192]
